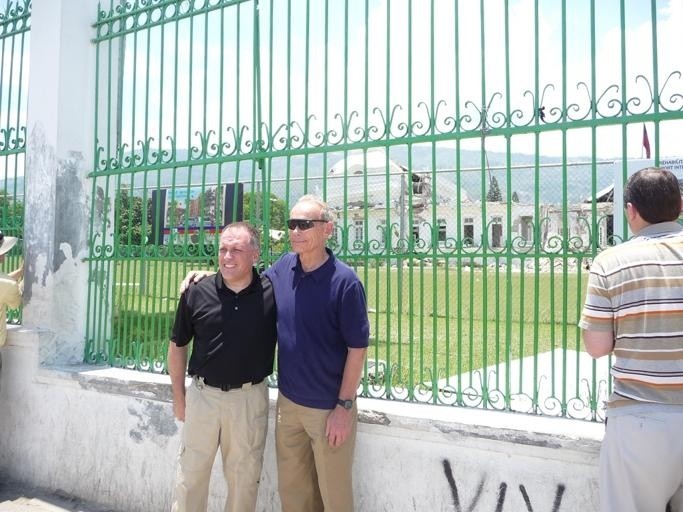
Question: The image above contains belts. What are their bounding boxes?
[196,376,265,390]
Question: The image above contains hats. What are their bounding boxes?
[0,232,17,255]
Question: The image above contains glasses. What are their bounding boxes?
[287,220,326,229]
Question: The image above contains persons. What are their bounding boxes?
[178,193,370,512]
[168,223,276,512]
[578,167,683,512]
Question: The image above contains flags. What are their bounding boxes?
[643,125,651,157]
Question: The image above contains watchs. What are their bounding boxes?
[336,392,357,410]
